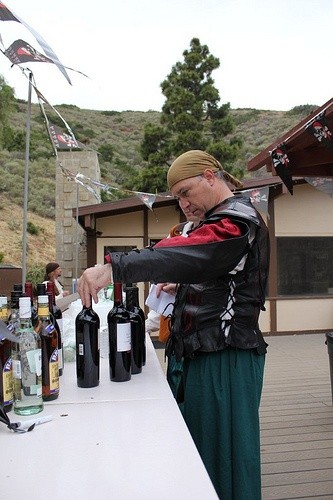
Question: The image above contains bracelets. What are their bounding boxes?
[110,267,114,285]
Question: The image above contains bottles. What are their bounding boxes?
[107,282,132,382]
[14,285,24,297]
[33,296,60,401]
[11,292,22,323]
[12,297,44,415]
[107,284,113,299]
[24,283,38,326]
[132,288,146,366]
[33,284,55,328]
[76,293,100,388]
[124,287,143,374]
[46,292,63,376]
[47,282,64,369]
[0,297,18,414]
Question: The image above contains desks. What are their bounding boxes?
[0,399,219,500]
[12,298,174,404]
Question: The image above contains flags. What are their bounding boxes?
[46,121,103,156]
[4,39,92,79]
[0,0,75,85]
[26,74,79,149]
[62,167,160,223]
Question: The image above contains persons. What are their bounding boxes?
[41,263,70,300]
